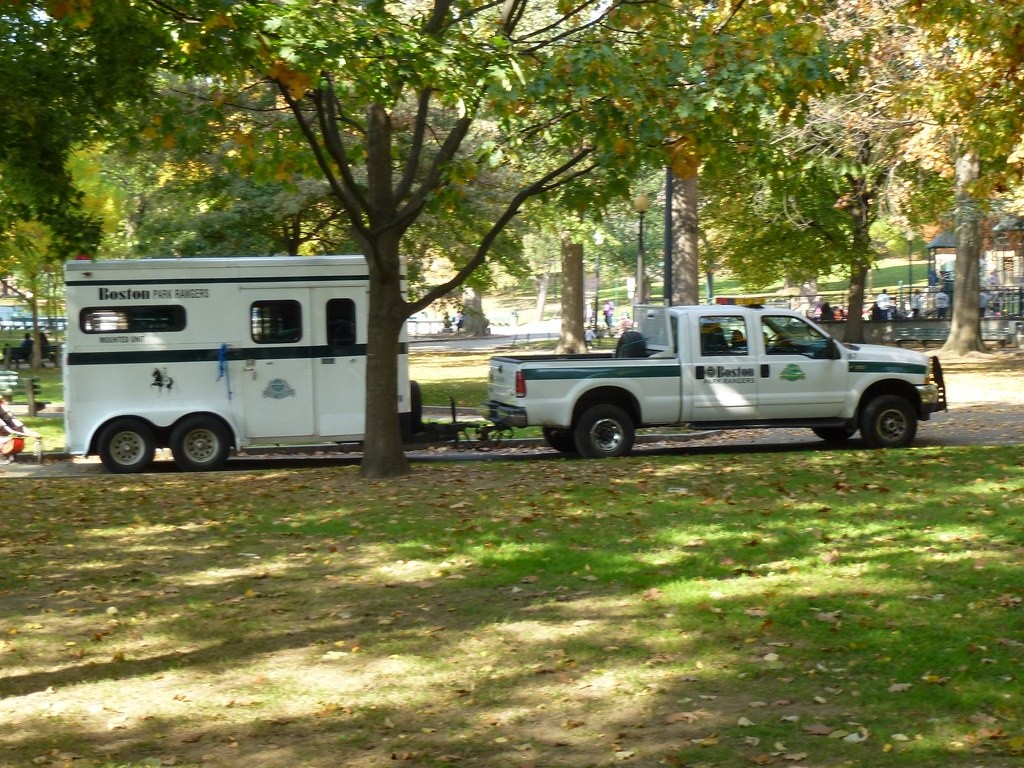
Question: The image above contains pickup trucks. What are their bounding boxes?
[482,294,948,460]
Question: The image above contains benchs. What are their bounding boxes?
[0,370,52,416]
[2,345,60,368]
[893,328,1009,348]
[11,316,67,330]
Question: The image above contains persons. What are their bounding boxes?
[980,288,1005,316]
[602,302,626,335]
[39,332,49,359]
[868,290,890,320]
[21,333,32,346]
[935,288,949,319]
[911,290,921,319]
[584,326,596,350]
[455,311,464,331]
[813,302,847,322]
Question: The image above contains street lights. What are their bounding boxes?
[634,191,649,306]
[593,230,604,330]
[905,228,914,306]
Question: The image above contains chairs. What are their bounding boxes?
[703,324,730,353]
